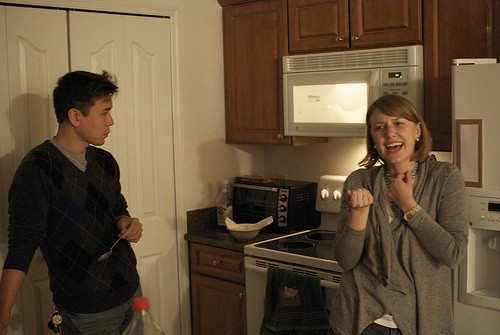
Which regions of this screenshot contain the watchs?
[404,205,422,221]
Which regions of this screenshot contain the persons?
[328,96,469,335]
[1,70,144,335]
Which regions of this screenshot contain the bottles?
[215,180,232,234]
[123,296,165,335]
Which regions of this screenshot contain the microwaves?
[281,45,423,138]
[231,178,321,235]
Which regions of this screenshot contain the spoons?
[97,236,121,261]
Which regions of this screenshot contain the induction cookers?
[244,175,349,272]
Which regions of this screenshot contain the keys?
[52,312,63,335]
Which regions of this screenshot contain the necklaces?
[385,164,419,215]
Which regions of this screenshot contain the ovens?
[244,256,341,335]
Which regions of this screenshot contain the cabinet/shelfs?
[188,243,246,335]
[218,0,500,152]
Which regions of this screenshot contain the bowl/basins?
[227,224,262,241]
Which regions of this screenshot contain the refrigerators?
[451,57,500,335]
